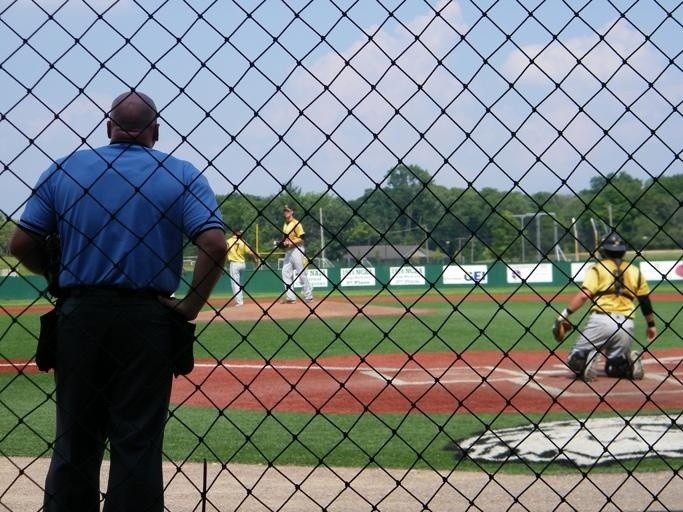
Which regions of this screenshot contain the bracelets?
[645,319,656,328]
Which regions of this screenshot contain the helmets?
[600,232,627,257]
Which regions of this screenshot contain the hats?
[232,229,244,234]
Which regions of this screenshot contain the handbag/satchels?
[173,318,196,377]
[36,308,54,372]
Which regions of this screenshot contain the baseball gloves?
[552,319,571,342]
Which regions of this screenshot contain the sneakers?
[626,349,645,380]
[280,299,314,304]
[232,301,245,306]
[582,348,603,383]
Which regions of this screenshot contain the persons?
[224,228,258,307]
[270,201,315,307]
[3,89,230,512]
[550,231,658,382]
